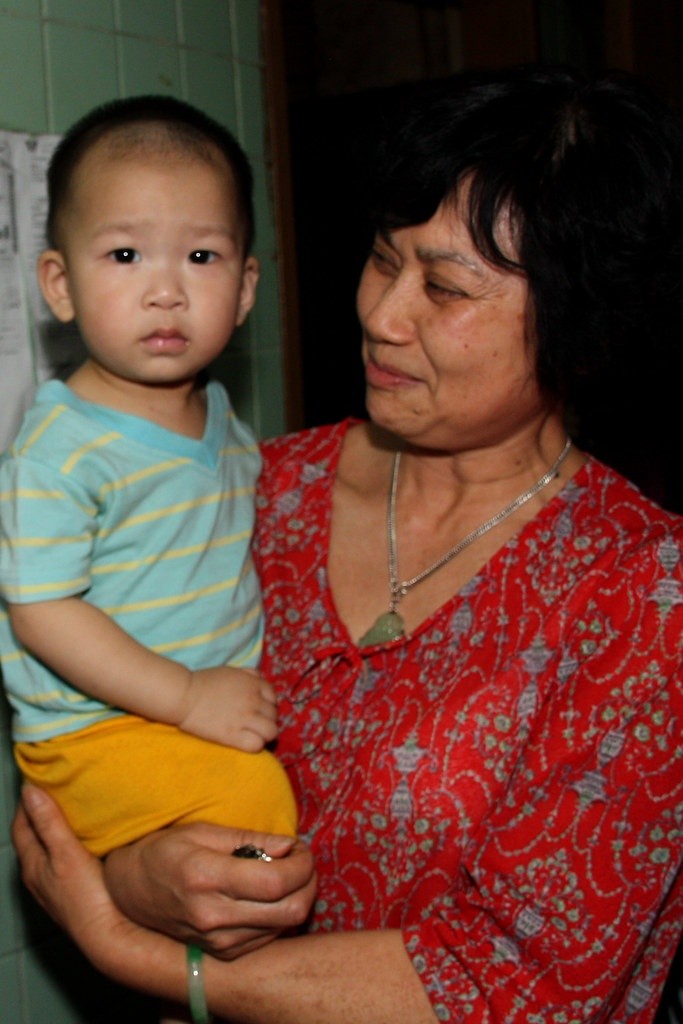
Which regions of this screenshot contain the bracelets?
[186,943,213,1024]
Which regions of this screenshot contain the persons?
[0,93,295,840]
[11,66,682,1024]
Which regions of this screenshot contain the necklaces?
[355,432,574,650]
